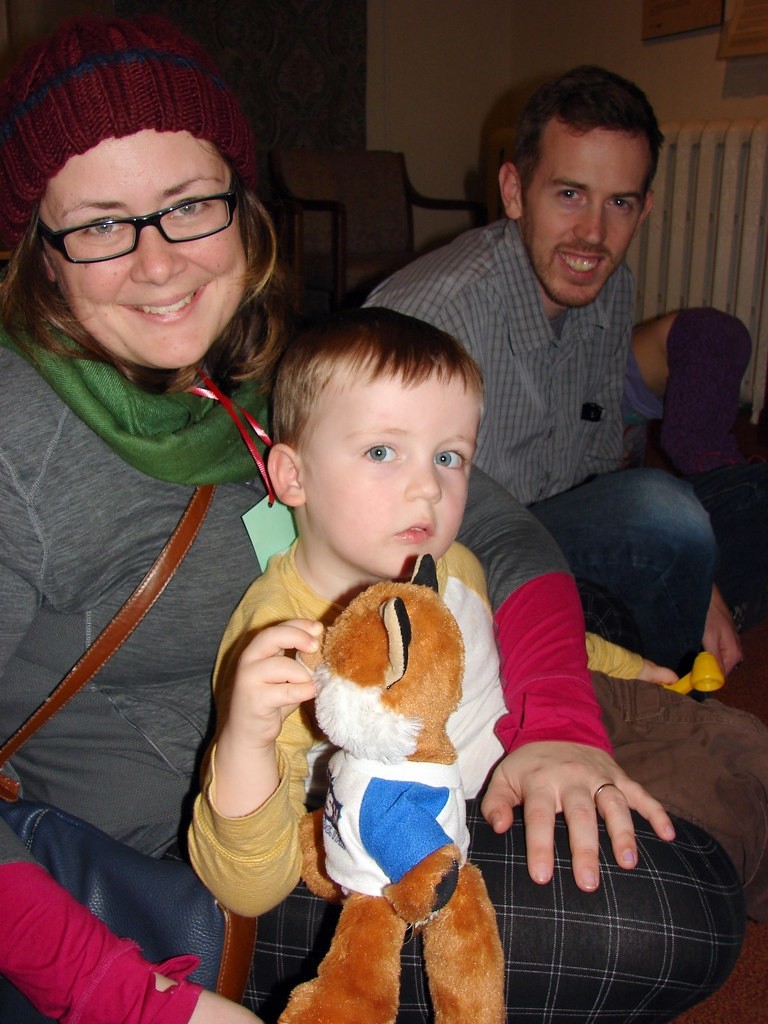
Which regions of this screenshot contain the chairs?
[268,145,488,325]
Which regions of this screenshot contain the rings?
[594,783,616,804]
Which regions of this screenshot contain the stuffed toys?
[276,553,506,1024]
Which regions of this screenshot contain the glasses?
[38,169,239,264]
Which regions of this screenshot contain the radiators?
[623,115,768,425]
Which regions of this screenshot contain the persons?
[362,64,753,703]
[188,307,679,917]
[0,4,745,1024]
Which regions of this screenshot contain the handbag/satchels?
[0,767,260,1002]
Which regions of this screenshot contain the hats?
[0,1,263,261]
